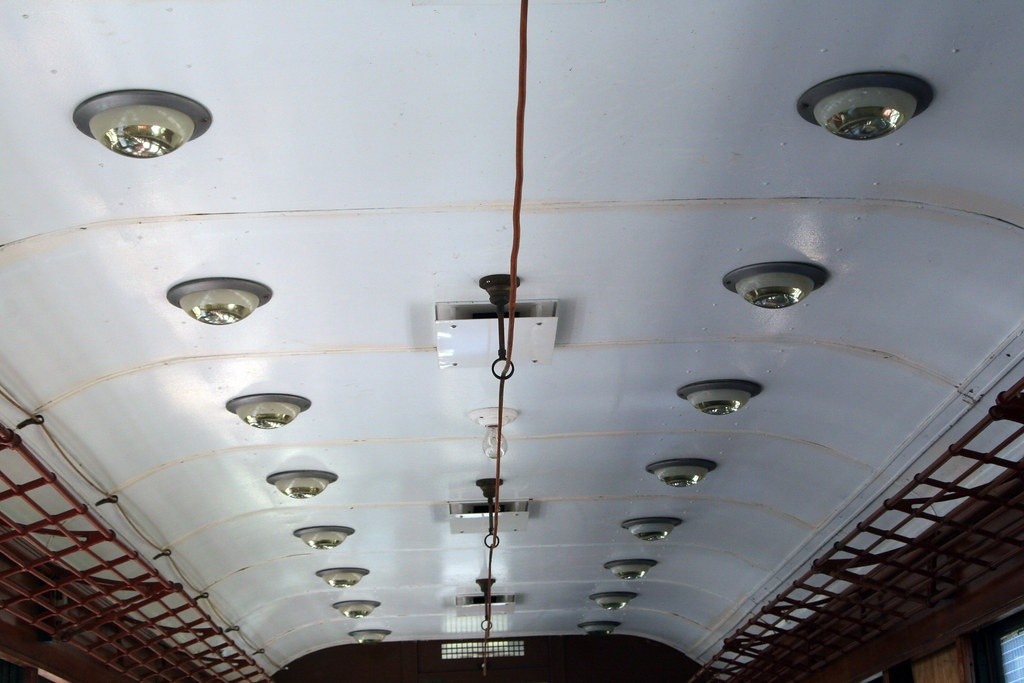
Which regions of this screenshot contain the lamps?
[620,517,684,541]
[453,594,516,616]
[432,299,560,370]
[796,71,934,141]
[348,629,392,644]
[677,378,764,416]
[166,276,273,326]
[722,262,831,310]
[315,567,370,589]
[645,458,718,488]
[266,470,339,499]
[226,393,312,430]
[448,500,532,533]
[589,591,639,611]
[293,525,356,551]
[604,558,659,580]
[578,621,622,637]
[332,599,381,619]
[72,88,212,159]
